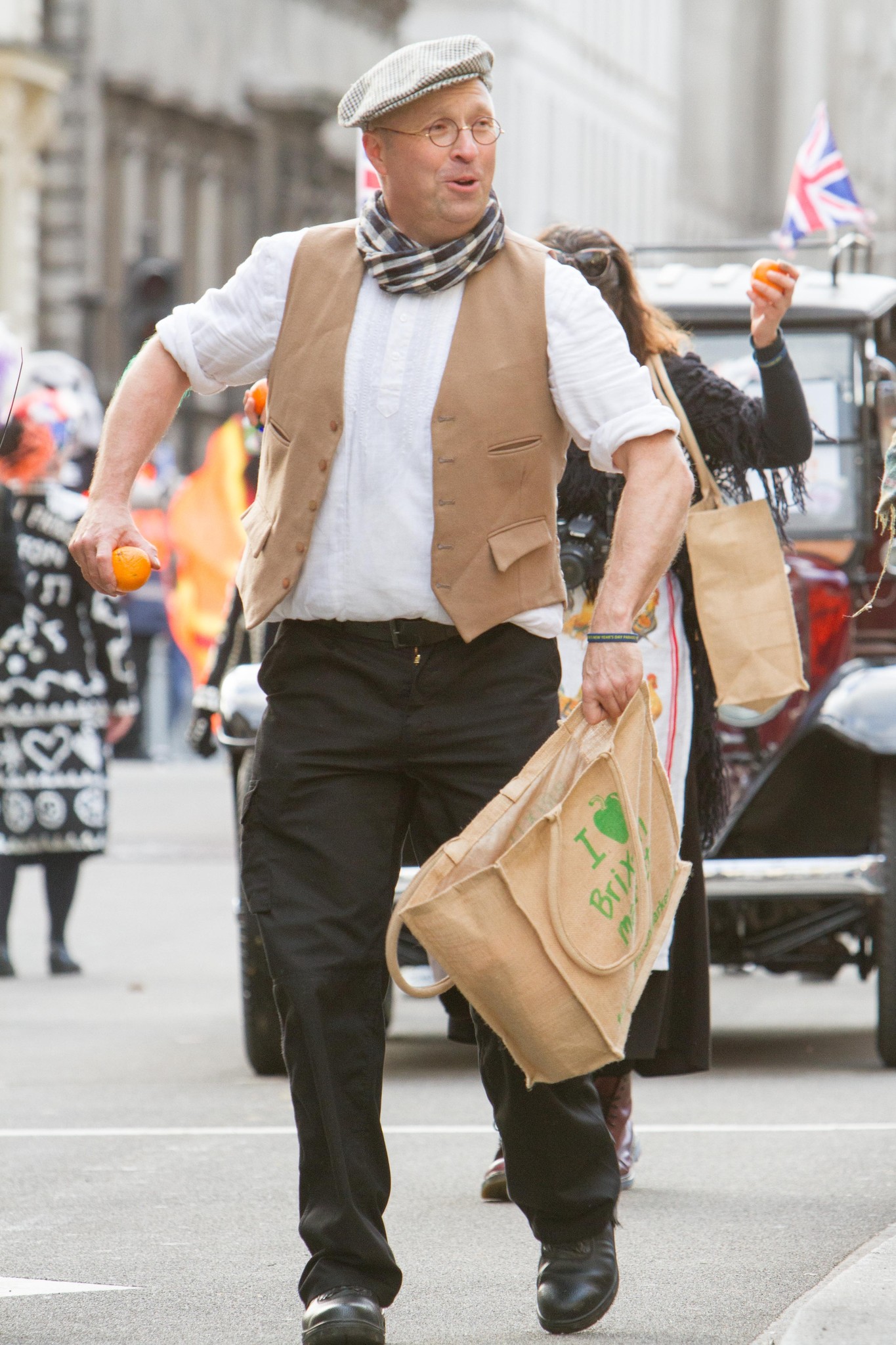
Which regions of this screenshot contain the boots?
[480,1145,512,1200]
[595,1066,641,1188]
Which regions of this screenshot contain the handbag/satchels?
[384,682,693,1089]
[646,354,810,715]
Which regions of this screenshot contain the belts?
[322,617,463,648]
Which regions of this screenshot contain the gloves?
[187,708,218,758]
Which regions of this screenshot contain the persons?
[481,228,813,1205]
[0,392,194,977]
[70,38,697,1345]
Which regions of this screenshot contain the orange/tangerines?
[751,258,787,298]
[112,546,151,592]
[249,377,269,414]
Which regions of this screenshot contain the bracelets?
[752,345,788,369]
[587,632,638,642]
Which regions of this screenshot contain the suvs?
[219,229,895,1081]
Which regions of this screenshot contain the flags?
[781,108,862,244]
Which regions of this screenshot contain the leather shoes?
[301,1285,386,1345]
[536,1207,620,1335]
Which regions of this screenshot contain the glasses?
[370,115,503,147]
[550,246,616,282]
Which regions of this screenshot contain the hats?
[0,395,67,486]
[339,34,496,130]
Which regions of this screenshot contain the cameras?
[555,513,609,591]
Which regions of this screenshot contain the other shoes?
[49,950,79,974]
[0,947,15,976]
